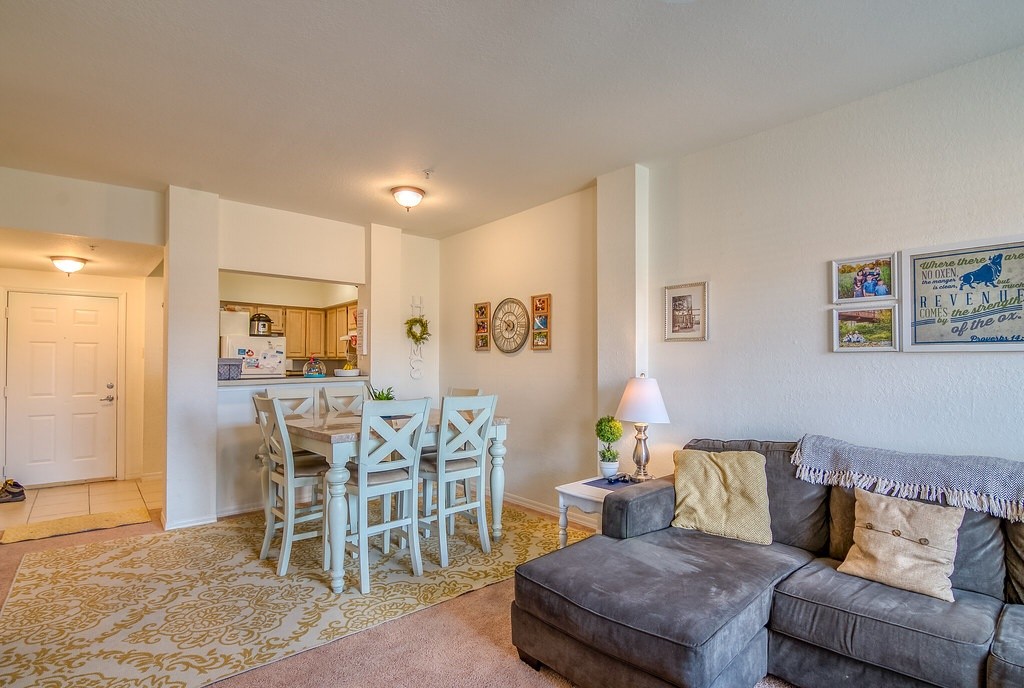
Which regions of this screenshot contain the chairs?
[423,386,483,538]
[346,397,431,594]
[322,385,367,417]
[418,394,498,567]
[252,395,330,576]
[266,387,322,524]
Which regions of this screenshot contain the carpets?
[0,507,152,544]
[0,485,596,688]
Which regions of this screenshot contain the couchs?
[511,434,828,688]
[767,434,1024,688]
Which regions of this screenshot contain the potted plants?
[371,386,397,419]
[595,416,623,477]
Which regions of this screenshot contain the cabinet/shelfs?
[285,310,325,360]
[326,302,357,359]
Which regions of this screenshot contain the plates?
[334,369,360,376]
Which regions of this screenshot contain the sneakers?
[0,480,26,502]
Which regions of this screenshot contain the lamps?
[391,186,425,211]
[49,257,87,277]
[614,372,671,482]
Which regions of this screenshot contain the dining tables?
[256,409,511,594]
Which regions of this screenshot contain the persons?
[843,330,864,343]
[853,266,888,297]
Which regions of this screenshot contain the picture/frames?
[832,304,899,351]
[664,281,707,342]
[531,294,551,350]
[902,234,1024,352]
[474,302,490,350]
[831,252,899,303]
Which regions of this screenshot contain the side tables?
[556,474,653,549]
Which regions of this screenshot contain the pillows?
[670,448,772,546]
[837,486,965,605]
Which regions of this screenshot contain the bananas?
[342,361,352,370]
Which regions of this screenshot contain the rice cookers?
[250,313,274,337]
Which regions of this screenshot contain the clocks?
[492,298,530,353]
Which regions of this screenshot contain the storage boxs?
[218,357,242,380]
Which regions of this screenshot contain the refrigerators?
[221,335,286,379]
